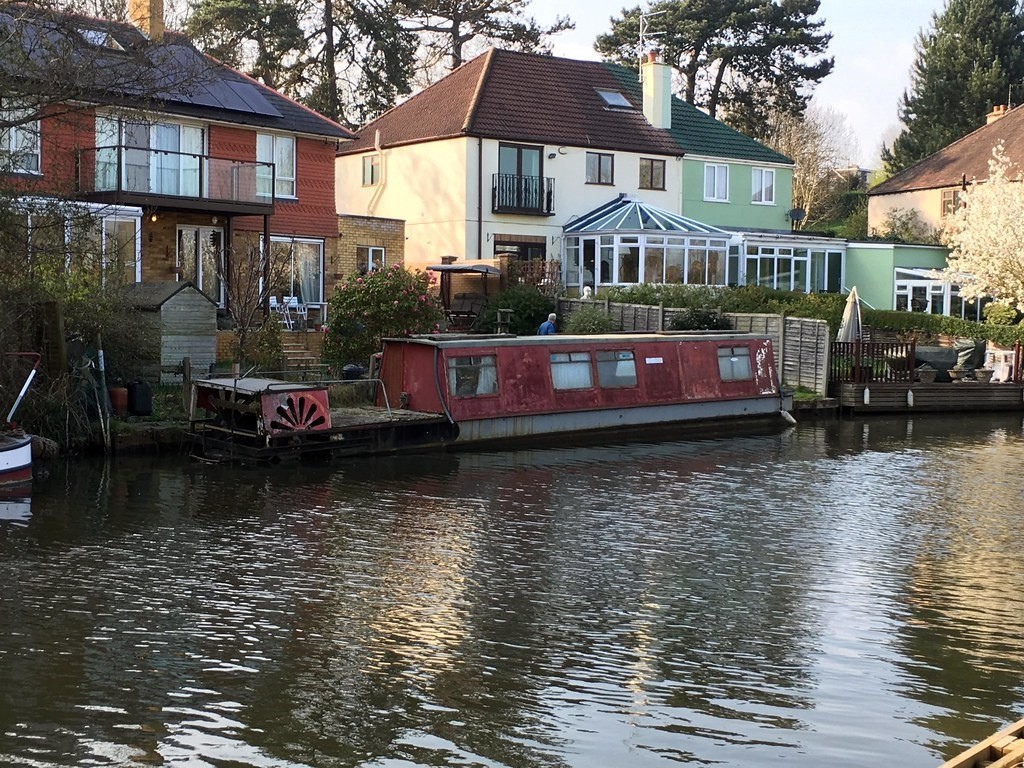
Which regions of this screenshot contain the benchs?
[443,293,485,328]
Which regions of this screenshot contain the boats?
[0,351,44,484]
[183,330,796,467]
[0,483,38,536]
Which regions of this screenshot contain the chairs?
[282,297,307,329]
[262,296,288,329]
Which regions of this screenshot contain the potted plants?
[974,366,995,383]
[947,364,969,383]
[915,365,938,383]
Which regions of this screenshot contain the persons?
[581,286,591,300]
[537,313,556,335]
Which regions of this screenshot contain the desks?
[301,302,331,324]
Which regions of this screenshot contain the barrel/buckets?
[852,364,873,382]
[314,324,321,331]
[109,377,152,421]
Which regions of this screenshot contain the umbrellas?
[833,285,858,365]
